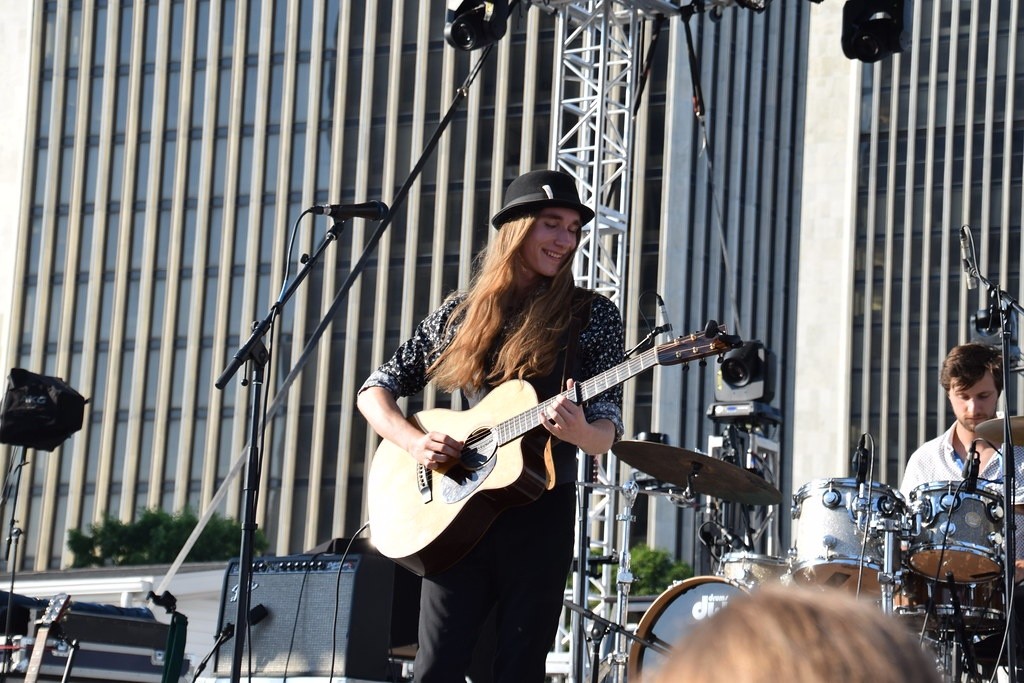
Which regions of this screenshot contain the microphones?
[959,229,977,290]
[308,200,389,221]
[222,604,267,642]
[658,295,674,344]
[852,435,866,473]
[713,521,743,550]
[962,441,978,478]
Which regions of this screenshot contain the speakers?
[213,553,395,682]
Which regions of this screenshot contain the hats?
[491,169,595,231]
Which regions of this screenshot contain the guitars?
[365,320,744,576]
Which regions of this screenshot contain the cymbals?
[1011,503,1024,515]
[969,415,1024,447]
[613,440,782,507]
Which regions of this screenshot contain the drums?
[791,479,907,593]
[903,479,1010,585]
[624,580,750,682]
[720,550,789,592]
[893,571,1006,634]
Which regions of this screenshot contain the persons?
[356,170,625,683]
[897,342,1024,683]
